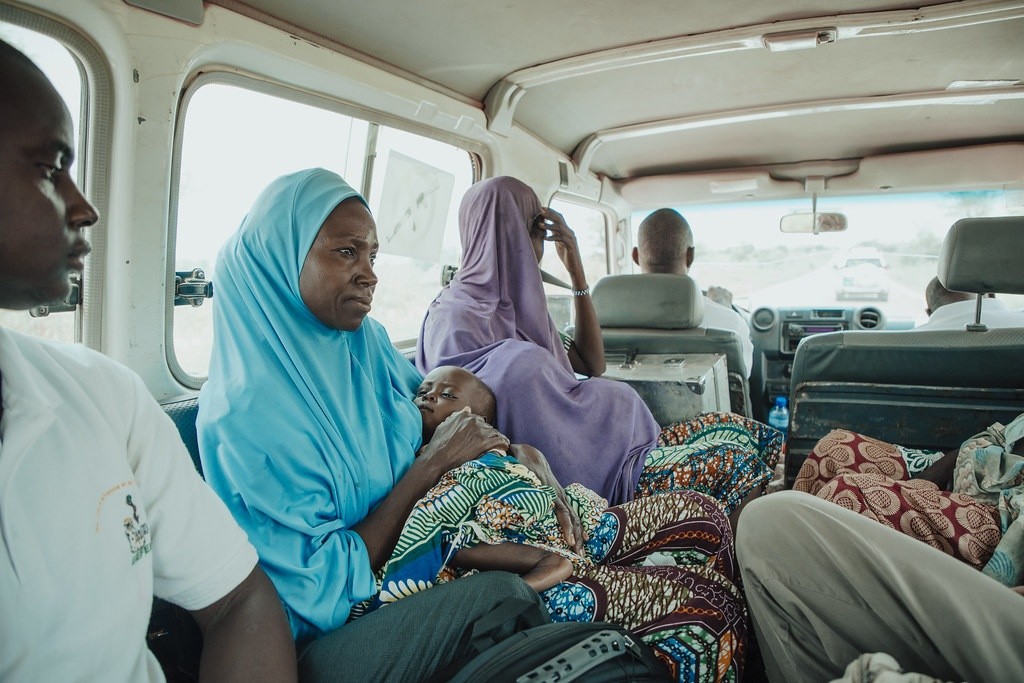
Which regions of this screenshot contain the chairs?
[562,272,756,416]
[786,215,1024,407]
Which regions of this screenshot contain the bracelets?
[571,284,589,296]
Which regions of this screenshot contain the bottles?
[767,397,789,442]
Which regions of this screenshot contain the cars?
[834,254,893,301]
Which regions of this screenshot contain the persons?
[0,39,547,683]
[734,272,1024,683]
[194,166,780,683]
